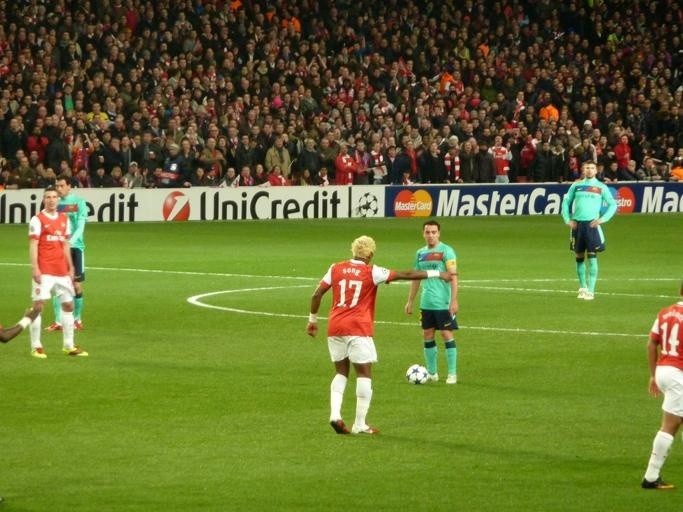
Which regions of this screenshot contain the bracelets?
[308,311,317,323]
[15,316,32,330]
[426,269,440,279]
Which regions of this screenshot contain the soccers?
[405,365,429,385]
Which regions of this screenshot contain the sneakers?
[577,288,594,300]
[427,373,439,382]
[642,476,675,489]
[63,346,87,357]
[32,348,47,359]
[330,418,349,433]
[445,374,457,385]
[351,423,378,435]
[47,320,82,331]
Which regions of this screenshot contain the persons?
[1,1,683,190]
[303,233,461,435]
[559,159,618,302]
[403,221,461,388]
[641,286,683,489]
[43,173,88,332]
[26,187,88,360]
[0,304,42,343]
